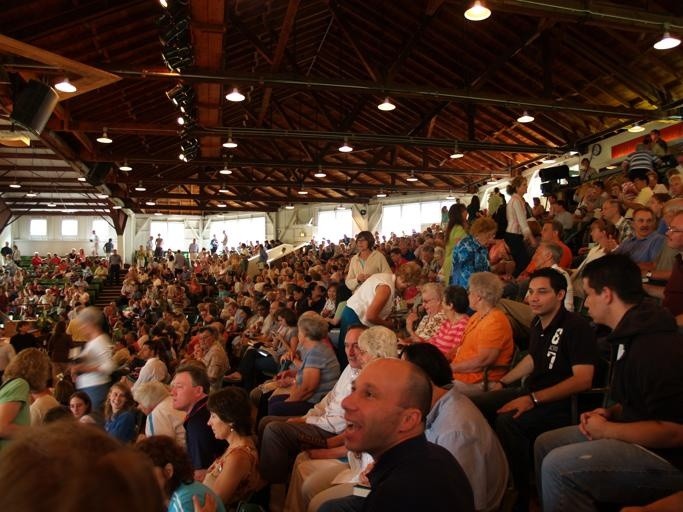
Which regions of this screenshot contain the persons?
[2,129,683,512]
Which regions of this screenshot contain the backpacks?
[493,202,509,240]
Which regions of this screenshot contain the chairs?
[186,314,199,327]
[569,341,612,424]
[240,349,276,385]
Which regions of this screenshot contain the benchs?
[19,256,106,305]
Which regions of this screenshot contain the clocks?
[592,144,601,156]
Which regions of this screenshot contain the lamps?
[10,181,21,189]
[653,31,680,51]
[155,0,199,164]
[517,110,535,124]
[406,174,419,181]
[54,74,156,207]
